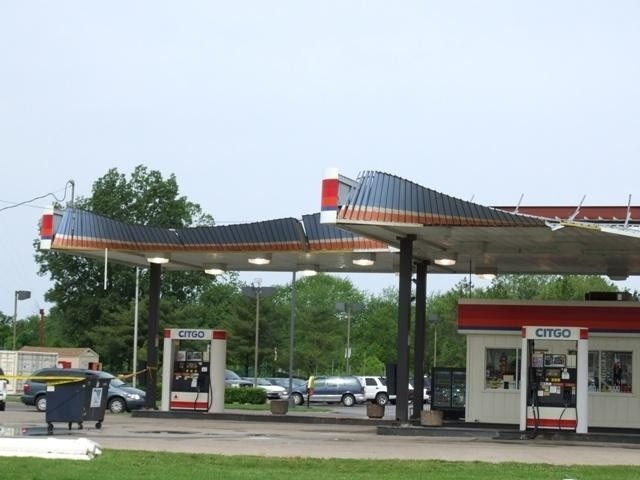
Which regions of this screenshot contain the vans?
[21,368,146,414]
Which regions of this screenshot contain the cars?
[485,368,519,389]
[588,370,632,391]
[433,384,466,407]
[224,369,431,406]
[0,368,9,411]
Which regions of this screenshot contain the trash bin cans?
[45,377,112,431]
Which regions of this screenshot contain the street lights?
[241,282,276,386]
[335,291,362,378]
[12,290,31,351]
[428,310,444,368]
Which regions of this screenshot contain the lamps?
[147,253,170,264]
[247,254,271,265]
[433,250,456,265]
[605,267,631,281]
[351,252,377,267]
[203,262,227,276]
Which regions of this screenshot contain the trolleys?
[46,370,116,431]
